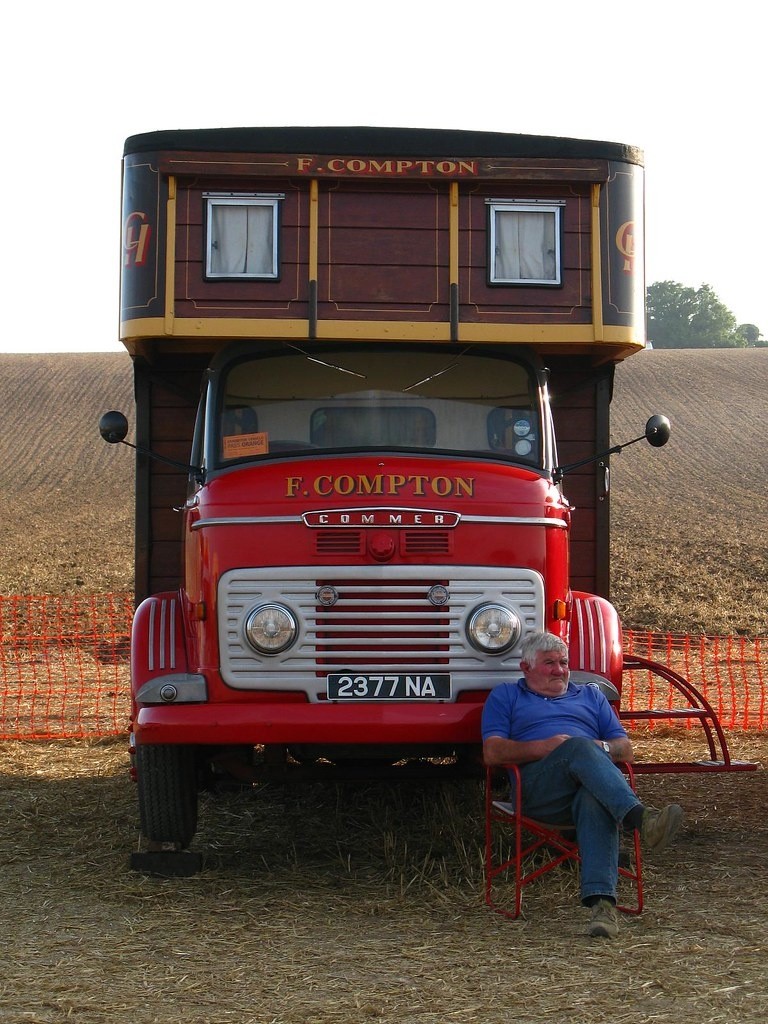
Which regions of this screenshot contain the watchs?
[600,740,610,753]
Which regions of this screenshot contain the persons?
[481,633,684,939]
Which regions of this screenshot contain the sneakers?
[589,899,619,939]
[641,804,685,855]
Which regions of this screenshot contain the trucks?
[100,125,672,851]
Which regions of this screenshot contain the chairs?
[484,760,644,920]
